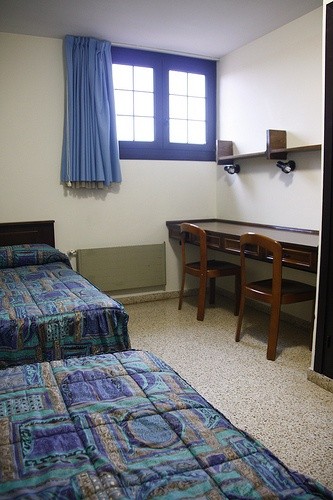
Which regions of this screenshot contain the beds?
[1,351,333,500]
[0,221,130,367]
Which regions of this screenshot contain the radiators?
[70,242,167,290]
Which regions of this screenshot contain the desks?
[166,218,319,272]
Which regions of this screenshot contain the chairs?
[178,223,241,321]
[236,233,316,361]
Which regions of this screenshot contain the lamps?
[277,160,296,174]
[224,164,240,174]
